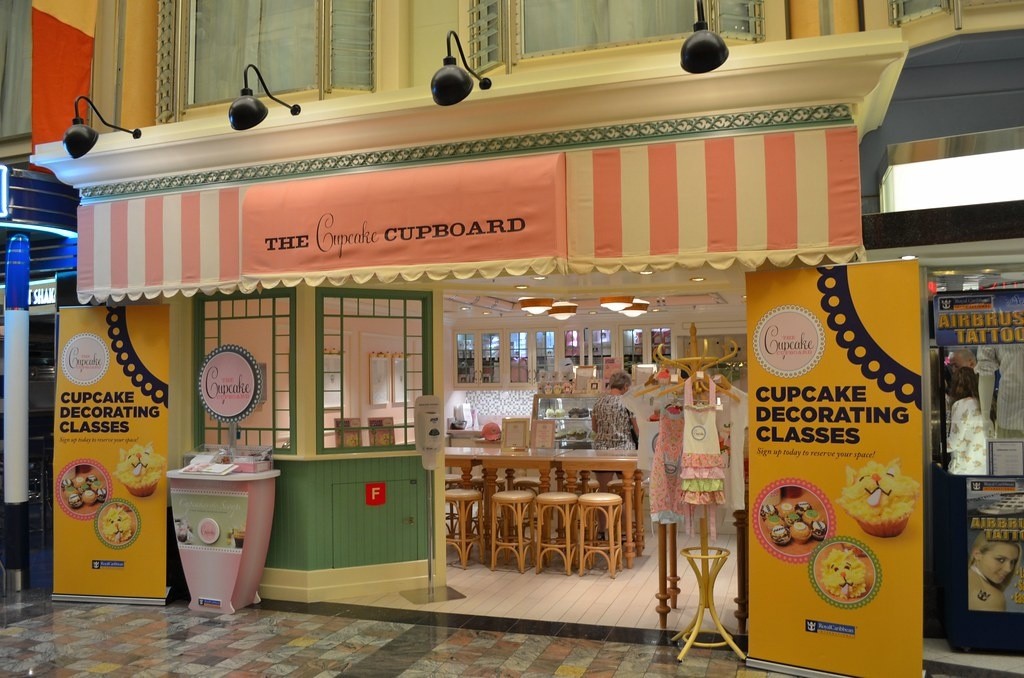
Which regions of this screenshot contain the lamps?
[517,295,553,315]
[680,0,728,73]
[598,294,633,311]
[618,297,651,317]
[63,95,142,158]
[228,64,301,130]
[547,301,578,320]
[429,29,492,106]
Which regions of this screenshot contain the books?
[183,462,235,473]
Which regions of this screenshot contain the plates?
[197,518,219,545]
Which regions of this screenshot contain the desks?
[557,447,636,568]
[442,444,571,568]
[165,469,281,612]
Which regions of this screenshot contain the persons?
[591,369,640,541]
[942,344,1024,475]
[968,530,1022,611]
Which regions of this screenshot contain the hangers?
[631,359,742,404]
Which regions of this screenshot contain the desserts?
[546,407,596,441]
[544,382,572,395]
[821,546,866,599]
[102,507,133,543]
[113,441,167,496]
[61,475,107,508]
[760,502,827,545]
[836,459,922,538]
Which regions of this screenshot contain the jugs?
[451,421,467,429]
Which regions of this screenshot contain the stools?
[443,475,645,579]
